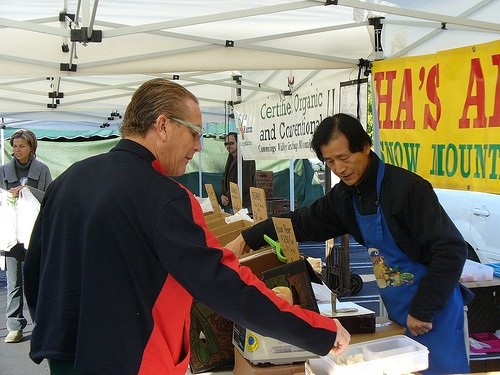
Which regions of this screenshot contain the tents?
[0,119,325,213]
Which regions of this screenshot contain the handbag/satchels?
[17,188,41,250]
[0,188,18,252]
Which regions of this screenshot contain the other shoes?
[4,330,23,343]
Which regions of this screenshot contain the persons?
[0,129,53,342]
[219,132,256,215]
[224,112,475,375]
[22,79,350,375]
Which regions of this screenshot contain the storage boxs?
[204,212,272,259]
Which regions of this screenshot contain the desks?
[233,316,424,375]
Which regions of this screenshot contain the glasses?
[224,142,236,146]
[169,116,204,139]
